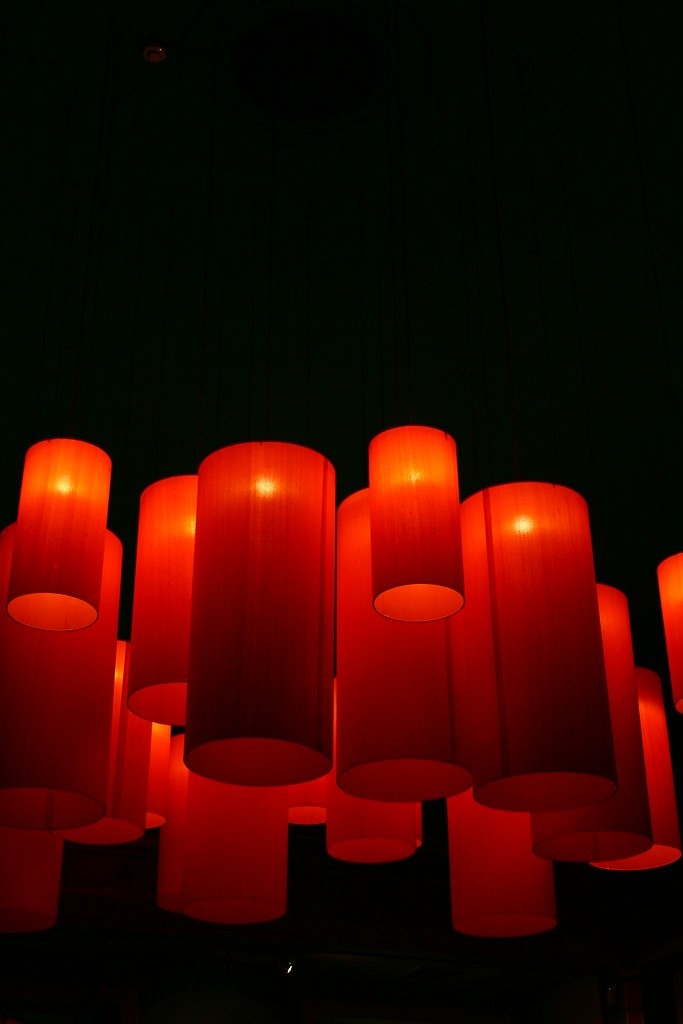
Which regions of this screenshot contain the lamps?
[1,428,682,941]
